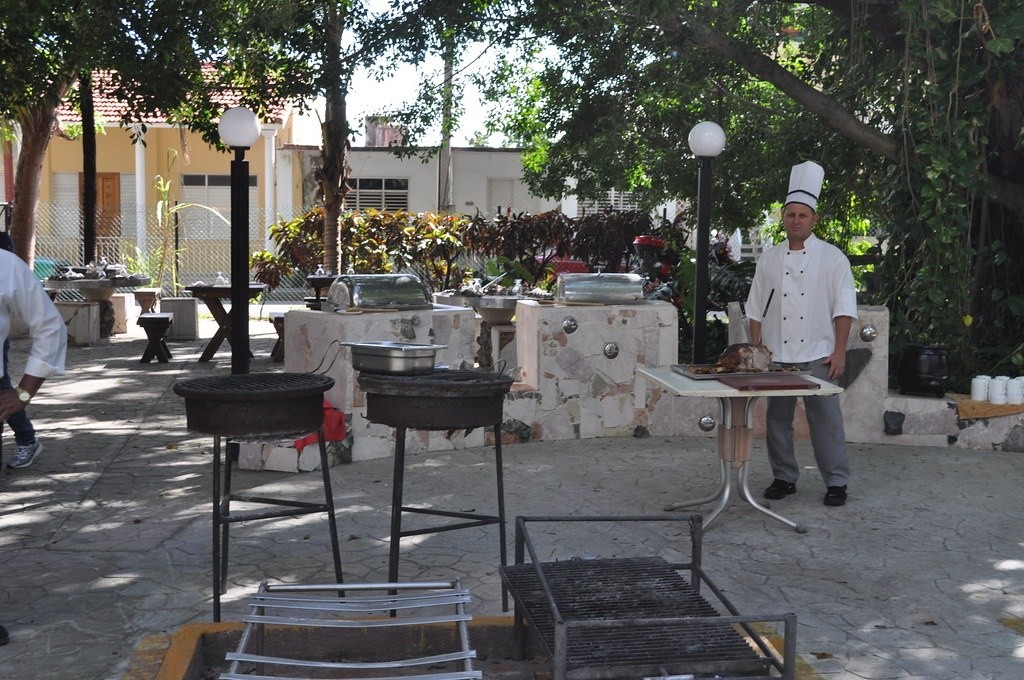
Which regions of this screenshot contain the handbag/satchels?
[295,400,345,452]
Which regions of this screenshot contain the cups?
[971,375,1024,404]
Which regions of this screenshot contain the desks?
[45,281,152,339]
[636,367,844,534]
[307,274,340,307]
[436,294,555,367]
[184,283,267,362]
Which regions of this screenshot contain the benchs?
[44,287,59,301]
[136,312,174,362]
[302,297,327,310]
[134,287,163,324]
[269,312,284,364]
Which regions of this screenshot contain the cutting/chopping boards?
[717,375,821,390]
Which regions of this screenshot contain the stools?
[55,300,102,345]
[111,293,135,333]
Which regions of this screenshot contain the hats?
[783,161,825,213]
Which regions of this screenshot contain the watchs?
[15,387,30,406]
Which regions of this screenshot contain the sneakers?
[764,479,796,499]
[7,439,43,469]
[824,484,847,506]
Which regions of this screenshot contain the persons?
[745,161,858,507]
[0,248,68,469]
